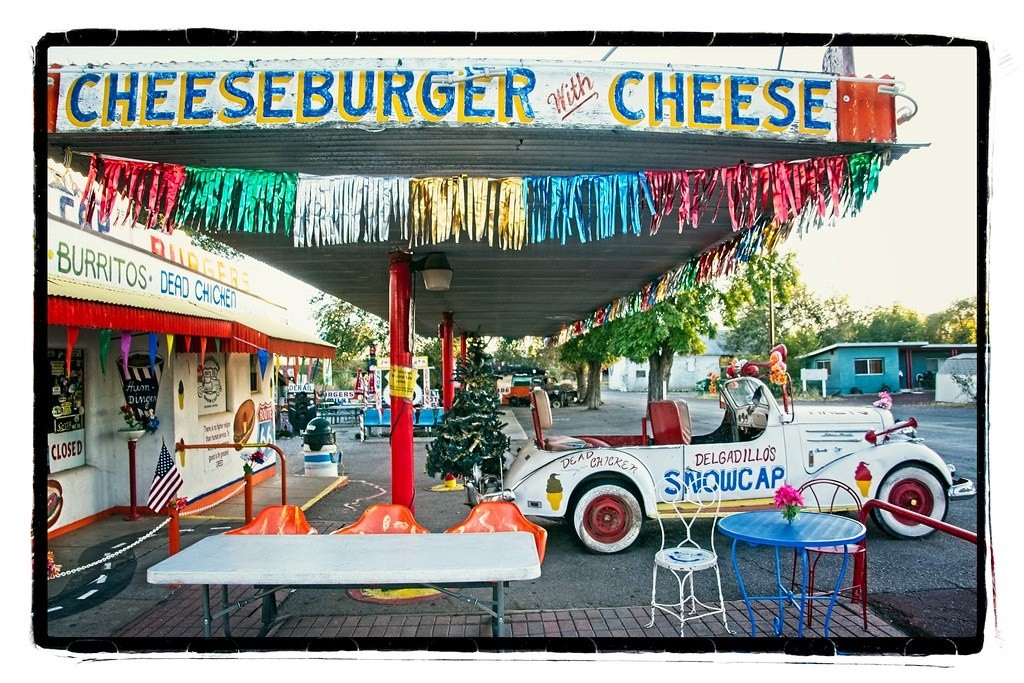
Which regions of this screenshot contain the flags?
[147,444,184,513]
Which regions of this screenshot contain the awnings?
[48,275,232,339]
[177,299,337,359]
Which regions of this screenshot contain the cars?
[466,344,977,556]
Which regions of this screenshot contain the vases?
[116,430,146,442]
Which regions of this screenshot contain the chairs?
[788,478,868,631]
[364,408,445,438]
[644,476,737,637]
[217,500,548,623]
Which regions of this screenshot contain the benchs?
[648,399,692,445]
[529,389,593,450]
[319,414,361,424]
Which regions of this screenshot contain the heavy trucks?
[505,376,580,409]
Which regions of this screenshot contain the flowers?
[241,450,265,474]
[769,362,788,386]
[774,485,805,524]
[119,404,155,431]
[166,496,188,511]
[706,357,738,394]
[873,390,892,410]
[47,551,62,579]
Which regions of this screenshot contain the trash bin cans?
[299,416,342,476]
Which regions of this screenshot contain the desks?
[147,533,541,637]
[717,510,863,637]
[317,402,365,427]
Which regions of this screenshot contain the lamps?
[412,253,454,291]
